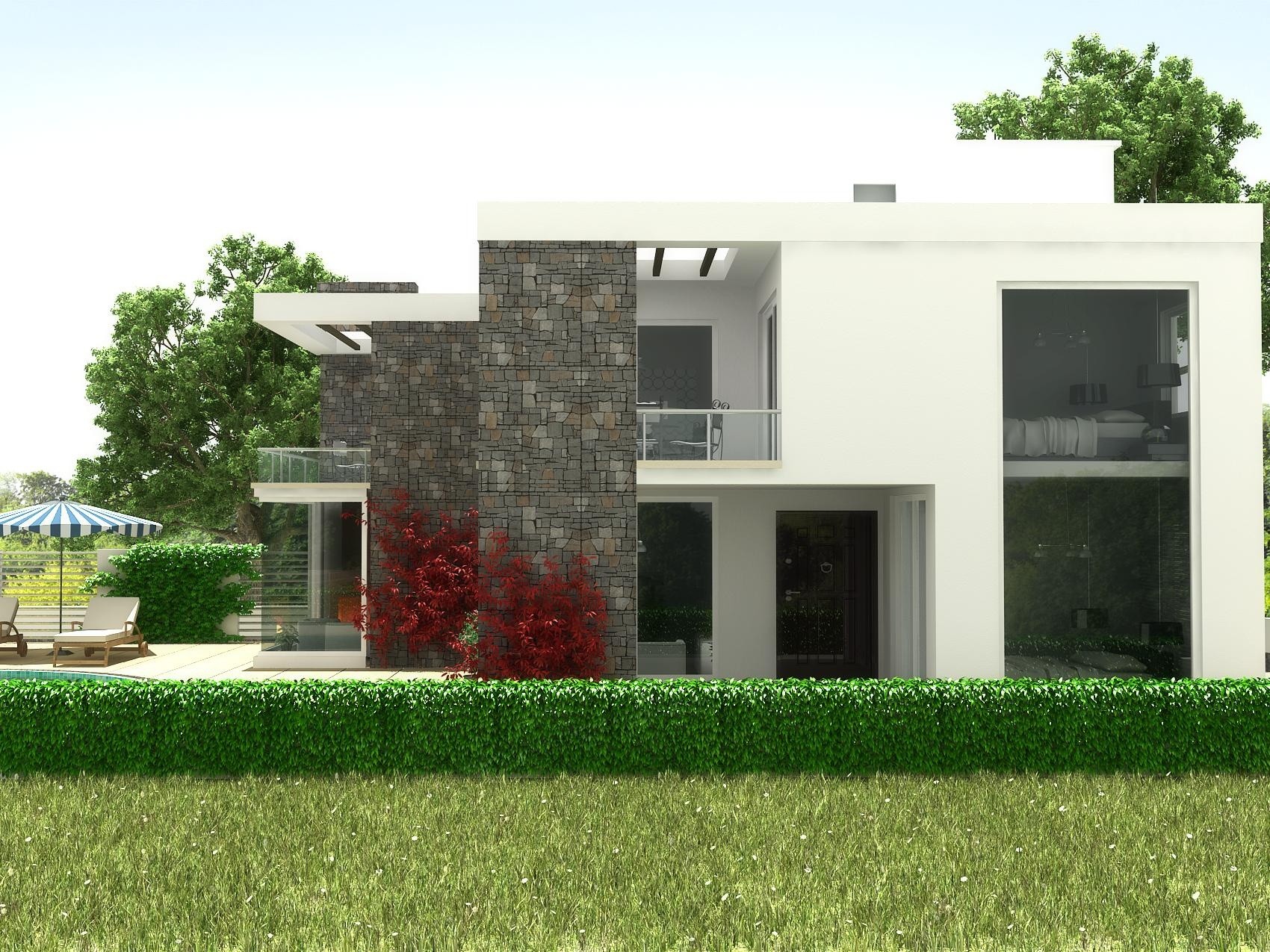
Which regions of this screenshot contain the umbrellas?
[0,501,164,649]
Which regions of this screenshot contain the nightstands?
[1147,442,1187,461]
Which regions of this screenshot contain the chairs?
[636,400,730,460]
[331,437,369,483]
[0,598,28,657]
[52,597,148,667]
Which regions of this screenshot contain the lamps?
[1137,291,1182,388]
[1034,291,1088,348]
[1035,484,1093,558]
[1069,290,1108,404]
[1142,478,1183,648]
[1072,480,1109,629]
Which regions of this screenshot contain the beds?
[1005,653,1152,679]
[1003,400,1170,459]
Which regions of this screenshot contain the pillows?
[1072,650,1147,672]
[1090,409,1144,422]
